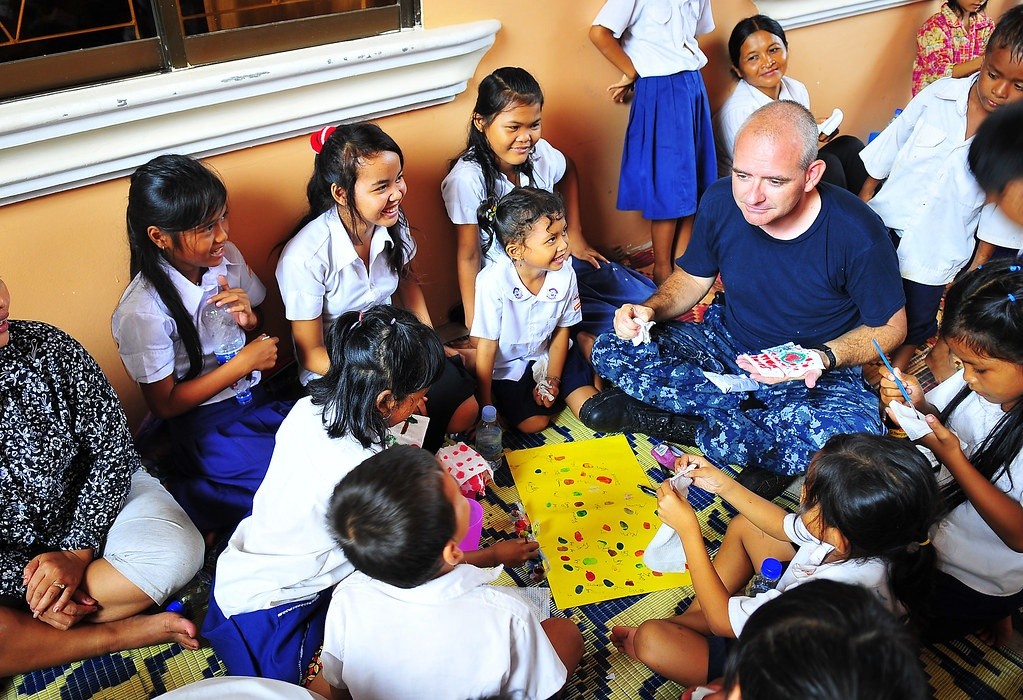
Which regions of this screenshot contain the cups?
[457,497,484,553]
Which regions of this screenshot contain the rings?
[54,583,65,589]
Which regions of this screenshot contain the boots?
[737,464,799,501]
[579,387,704,446]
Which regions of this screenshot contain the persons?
[711,15,882,198]
[610,433,939,694]
[469,185,599,434]
[110,153,291,514]
[0,277,206,677]
[275,123,479,435]
[912,0,996,99]
[318,445,584,700]
[725,579,924,700]
[879,257,1023,643]
[588,0,717,285]
[858,3,1023,373]
[440,66,657,339]
[579,99,908,477]
[201,303,539,695]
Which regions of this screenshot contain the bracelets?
[815,345,836,370]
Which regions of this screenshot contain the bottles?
[744,556,782,598]
[474,405,503,472]
[164,526,236,618]
[200,302,253,407]
[886,108,903,128]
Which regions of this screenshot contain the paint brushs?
[872,338,921,419]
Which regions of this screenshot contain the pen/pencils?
[637,484,656,497]
[523,536,530,545]
